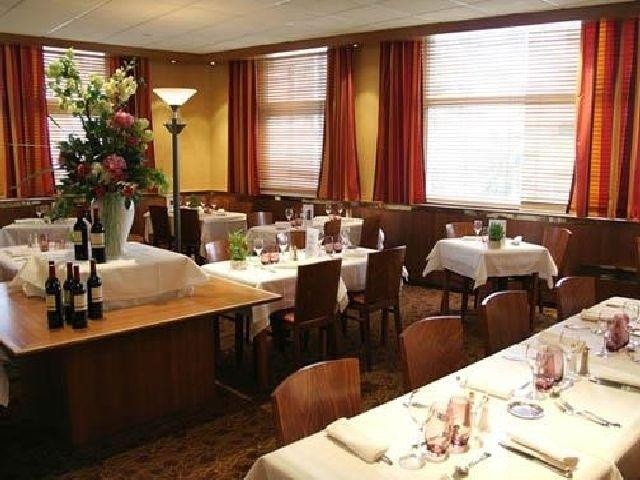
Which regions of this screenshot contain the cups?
[425,412,451,460]
[526,339,547,399]
[261,249,270,264]
[36,205,48,221]
[623,302,640,353]
[547,348,564,379]
[604,320,618,352]
[292,249,298,261]
[279,236,288,260]
[325,236,333,253]
[408,393,436,469]
[295,218,301,229]
[338,208,342,217]
[28,232,38,249]
[270,245,279,262]
[598,309,615,358]
[326,205,332,216]
[446,399,470,452]
[474,220,482,241]
[536,353,554,387]
[483,226,489,243]
[613,313,630,345]
[286,208,294,227]
[334,235,342,253]
[290,245,297,257]
[254,237,264,265]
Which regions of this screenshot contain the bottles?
[40,233,50,254]
[63,261,74,326]
[86,261,103,321]
[73,206,88,261]
[45,261,65,330]
[90,208,107,264]
[70,266,87,330]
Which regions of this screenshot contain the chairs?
[251,258,342,382]
[339,247,405,352]
[272,358,361,446]
[360,214,381,251]
[481,290,531,357]
[181,208,205,265]
[323,219,341,245]
[286,230,307,251]
[247,211,274,229]
[399,316,466,393]
[554,275,595,321]
[229,199,250,211]
[445,220,481,316]
[206,238,231,262]
[525,226,573,315]
[148,204,175,252]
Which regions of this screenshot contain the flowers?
[17,51,171,222]
[485,218,504,241]
[226,227,246,260]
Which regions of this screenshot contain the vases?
[488,236,501,249]
[100,192,134,264]
[231,257,245,269]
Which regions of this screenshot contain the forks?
[557,399,620,429]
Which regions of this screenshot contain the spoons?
[454,453,496,476]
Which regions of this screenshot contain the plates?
[506,400,545,420]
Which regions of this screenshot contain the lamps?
[153,87,197,106]
